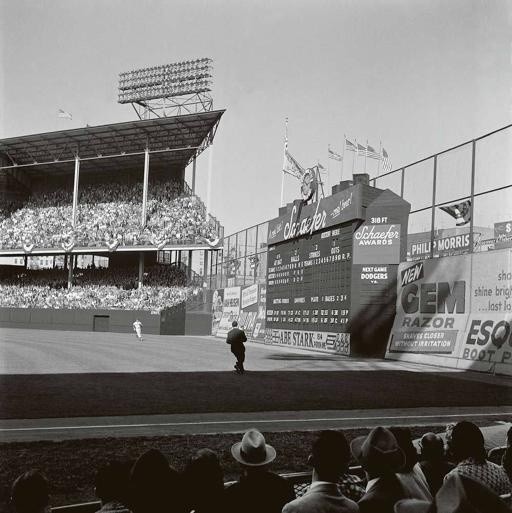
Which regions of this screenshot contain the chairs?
[487,445,508,465]
[495,493,512,513]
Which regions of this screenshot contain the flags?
[365,143,380,159]
[354,141,367,156]
[379,146,392,171]
[327,145,342,162]
[344,138,357,152]
[281,151,306,178]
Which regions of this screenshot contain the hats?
[350,428,407,474]
[232,431,276,467]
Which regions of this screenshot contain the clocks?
[298,168,315,201]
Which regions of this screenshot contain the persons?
[132,319,145,341]
[90,416,512,513]
[0,165,225,313]
[225,320,247,374]
[9,469,53,512]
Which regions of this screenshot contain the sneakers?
[234,365,244,374]
